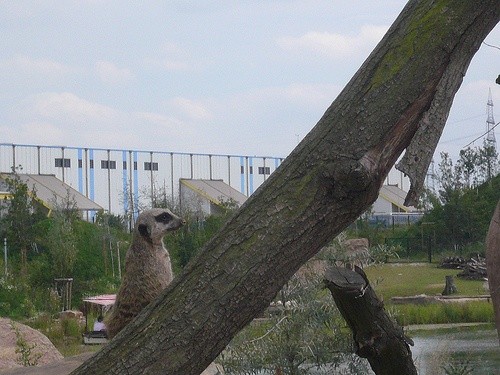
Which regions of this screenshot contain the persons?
[93,315,106,331]
